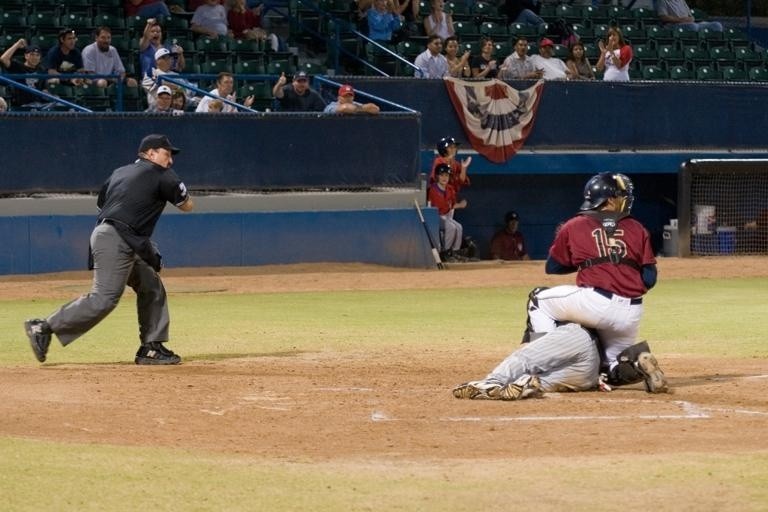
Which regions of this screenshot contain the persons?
[24,132,193,364]
[651,0,697,25]
[356,1,635,82]
[452,323,603,403]
[490,211,530,262]
[0,0,382,114]
[527,171,669,392]
[427,136,473,263]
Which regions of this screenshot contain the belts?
[594,286,641,305]
[98,218,114,224]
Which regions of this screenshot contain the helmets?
[437,135,461,156]
[435,163,452,175]
[580,172,635,215]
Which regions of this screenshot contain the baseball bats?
[414,198,444,270]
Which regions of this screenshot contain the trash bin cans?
[663,225,678,257]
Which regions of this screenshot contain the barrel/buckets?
[661,224,679,257]
[717,226,739,254]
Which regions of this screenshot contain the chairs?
[0,2,766,118]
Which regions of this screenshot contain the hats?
[338,85,355,96]
[156,85,171,96]
[539,38,554,48]
[506,212,519,222]
[294,71,308,83]
[155,48,178,61]
[26,45,42,55]
[139,135,181,155]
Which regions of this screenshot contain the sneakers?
[24,318,51,362]
[637,351,669,394]
[452,375,542,400]
[135,345,180,365]
